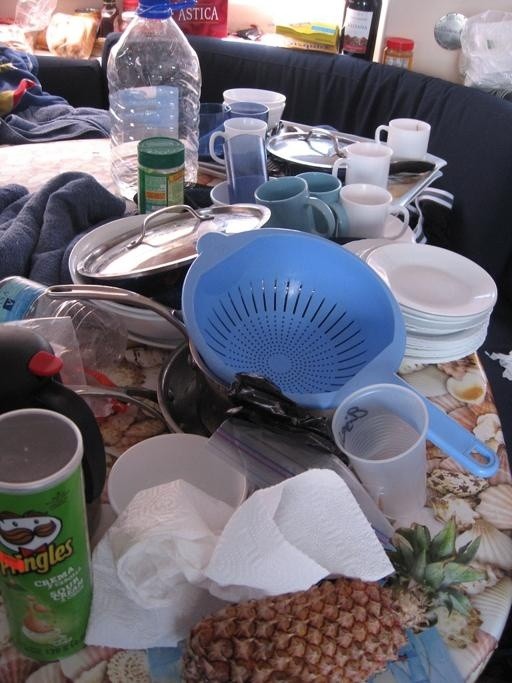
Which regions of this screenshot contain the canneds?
[119,11,137,32]
[381,38,413,72]
[74,8,100,38]
[137,137,186,214]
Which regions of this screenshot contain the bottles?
[105,0,203,202]
[101,0,121,37]
[1,273,129,372]
[338,0,382,61]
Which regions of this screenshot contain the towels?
[84,469,396,648]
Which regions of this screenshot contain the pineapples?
[181,518,486,683]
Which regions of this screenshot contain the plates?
[358,215,417,245]
[105,432,246,532]
[333,236,498,363]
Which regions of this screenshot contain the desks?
[1,139,511,682]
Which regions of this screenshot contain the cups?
[196,100,222,136]
[221,100,432,241]
[328,382,431,522]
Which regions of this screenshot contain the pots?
[68,203,272,349]
[46,282,389,483]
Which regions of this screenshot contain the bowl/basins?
[221,86,287,134]
[208,175,280,208]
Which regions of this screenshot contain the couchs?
[102,31,512,471]
[0,53,102,145]
